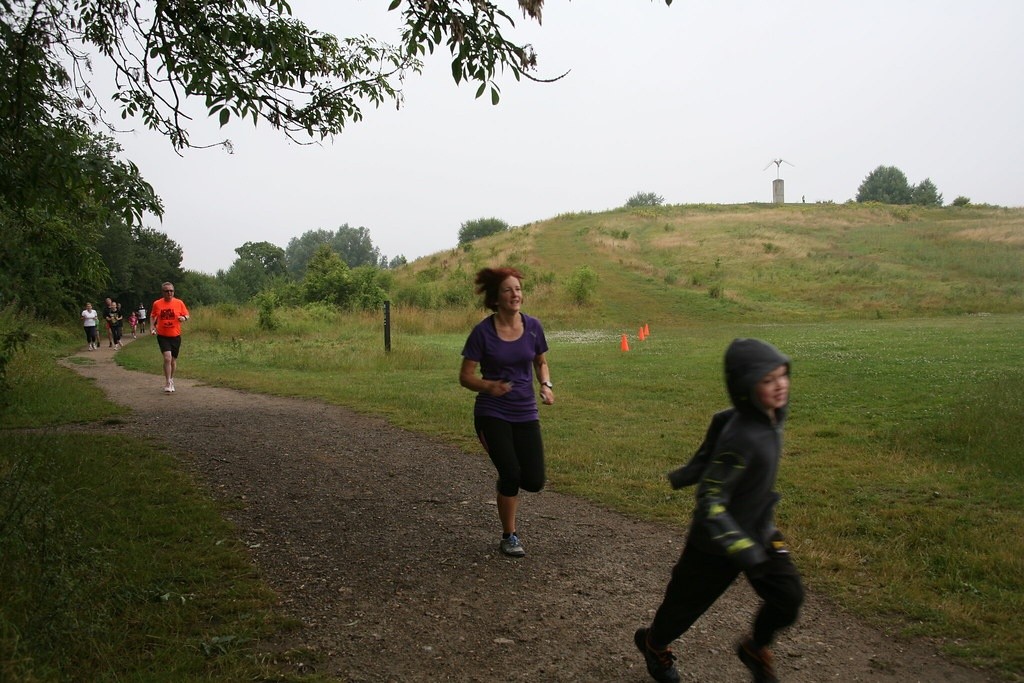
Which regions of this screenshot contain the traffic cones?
[639,326,645,341]
[644,322,650,337]
[620,333,629,352]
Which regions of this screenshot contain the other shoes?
[97,342,100,347]
[114,344,119,350]
[92,344,97,349]
[134,335,136,338]
[88,345,93,351]
[118,340,123,347]
[109,342,112,347]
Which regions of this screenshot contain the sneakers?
[738,636,780,683]
[501,532,525,557]
[634,627,679,683]
[164,379,175,392]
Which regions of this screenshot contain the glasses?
[163,289,175,292]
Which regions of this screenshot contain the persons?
[91,306,100,347]
[150,282,190,393]
[102,298,113,347]
[459,266,555,556]
[81,302,98,351]
[634,339,807,683]
[105,302,123,350]
[136,302,148,333]
[128,312,138,338]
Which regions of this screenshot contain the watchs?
[184,315,188,321]
[541,382,553,390]
